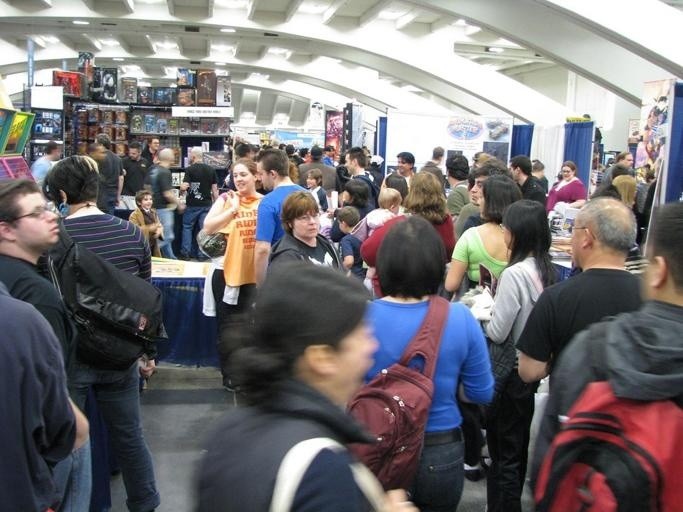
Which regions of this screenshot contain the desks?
[149,253,217,370]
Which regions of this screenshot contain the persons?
[29,135,655,478]
[0,282,92,512]
[527,200,682,512]
[192,261,419,512]
[344,217,494,512]
[39,154,160,512]
[476,200,562,512]
[0,177,92,512]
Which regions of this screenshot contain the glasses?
[293,213,319,221]
[567,225,597,241]
[7,201,57,224]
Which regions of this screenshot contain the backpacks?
[531,378,683,512]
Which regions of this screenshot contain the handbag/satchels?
[46,241,164,371]
[195,226,229,259]
[343,362,437,498]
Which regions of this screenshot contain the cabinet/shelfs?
[20,105,65,165]
[73,103,234,220]
